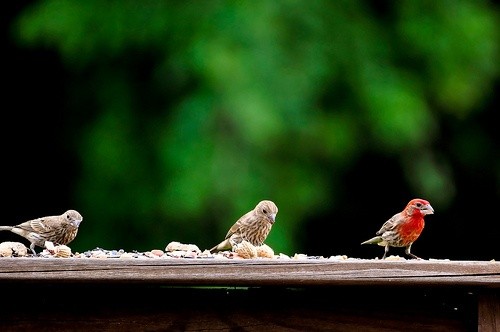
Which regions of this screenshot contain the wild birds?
[361,199,435,261]
[0,210,83,256]
[208,200,278,252]
